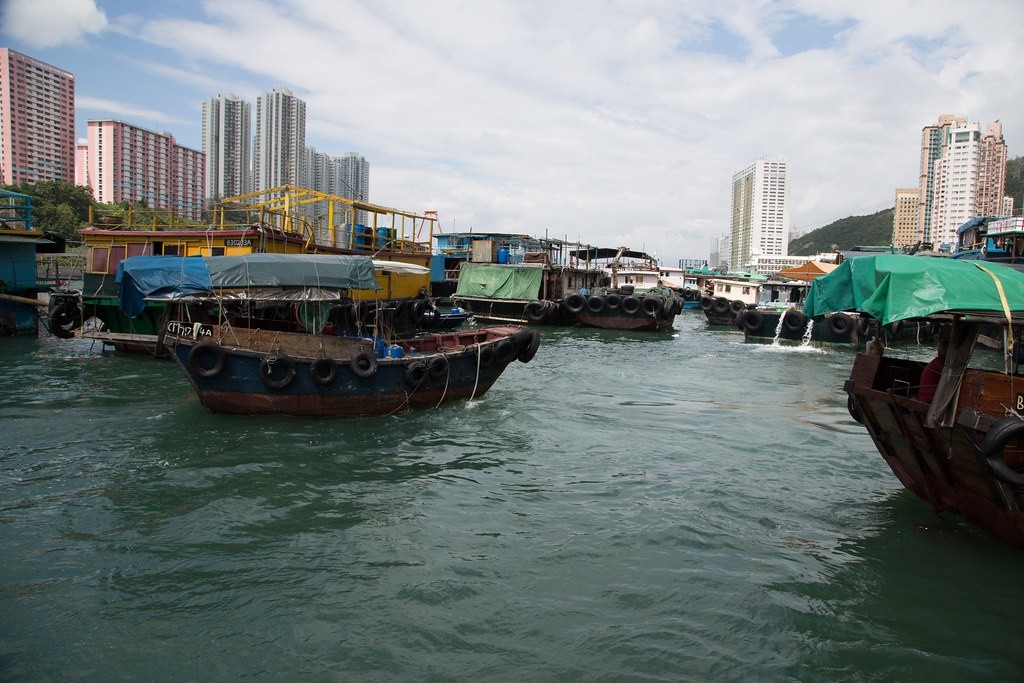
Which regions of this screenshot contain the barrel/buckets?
[373,338,386,360]
[497,248,507,264]
[452,307,460,314]
[381,342,405,357]
[334,223,389,250]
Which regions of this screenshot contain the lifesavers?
[519,328,541,365]
[428,356,450,380]
[259,352,296,387]
[527,300,546,321]
[493,340,514,367]
[187,342,227,378]
[310,355,338,385]
[783,310,805,332]
[668,286,701,300]
[699,298,744,314]
[741,310,764,331]
[982,418,1024,487]
[565,292,662,316]
[857,317,905,340]
[352,351,381,378]
[477,346,493,368]
[407,362,428,386]
[50,301,84,339]
[828,313,853,335]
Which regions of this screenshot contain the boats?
[0,184,540,417]
[805,255,1024,549]
[428,213,1024,353]
